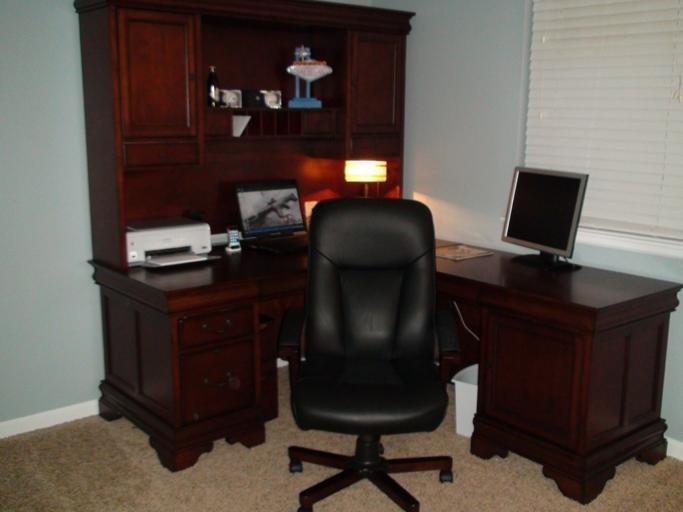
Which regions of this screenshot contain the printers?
[124,216,212,269]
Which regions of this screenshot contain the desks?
[98,229,340,476]
[450,241,670,504]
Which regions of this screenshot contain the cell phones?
[226,227,240,248]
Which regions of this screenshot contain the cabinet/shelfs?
[206,3,344,161]
[76,5,212,218]
[342,2,403,160]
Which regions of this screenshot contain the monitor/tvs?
[233,178,306,255]
[502,167,588,267]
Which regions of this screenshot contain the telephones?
[225,225,242,255]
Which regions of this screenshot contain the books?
[434,243,494,261]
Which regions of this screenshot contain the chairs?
[276,198,467,511]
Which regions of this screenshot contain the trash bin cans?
[451,364,479,438]
